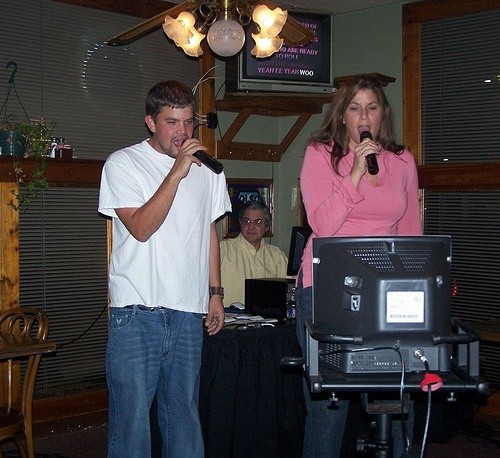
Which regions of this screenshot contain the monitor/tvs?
[310,235,453,374]
[236,10,335,94]
[287,226,313,276]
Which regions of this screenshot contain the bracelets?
[209,286,224,300]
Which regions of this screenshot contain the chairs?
[0,307,49,458]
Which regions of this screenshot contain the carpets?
[0,423,107,458]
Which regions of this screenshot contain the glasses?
[240,218,268,227]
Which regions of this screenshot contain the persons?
[296,75,424,458]
[98,81,234,458]
[220,200,288,308]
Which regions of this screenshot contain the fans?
[105,0,315,47]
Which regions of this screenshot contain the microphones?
[360,130,379,175]
[182,140,224,175]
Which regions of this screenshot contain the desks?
[150,310,490,458]
[0,332,56,360]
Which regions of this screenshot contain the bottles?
[50,136,71,157]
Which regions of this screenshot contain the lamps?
[163,0,288,58]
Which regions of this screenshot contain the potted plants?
[0,114,73,211]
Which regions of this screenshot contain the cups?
[55,146,73,158]
[1,130,23,156]
